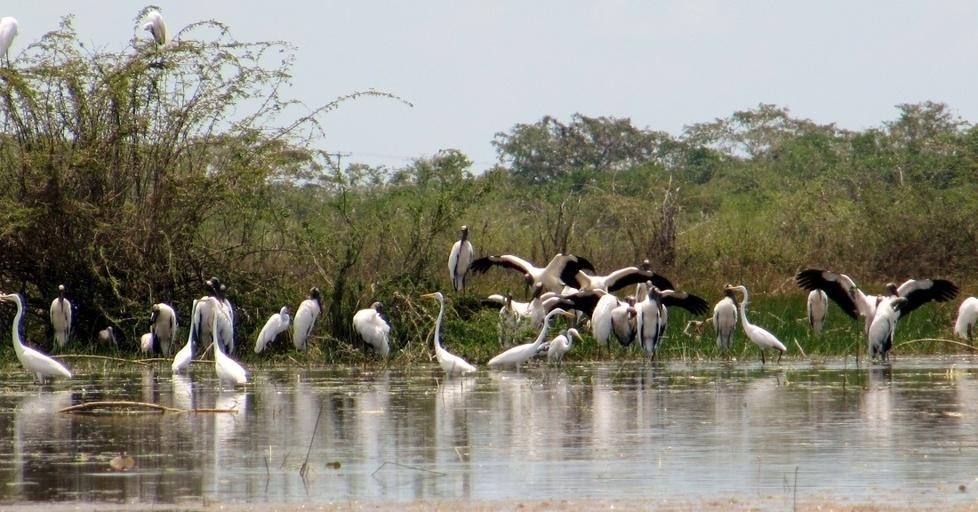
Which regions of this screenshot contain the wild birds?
[0,223,972,386]
